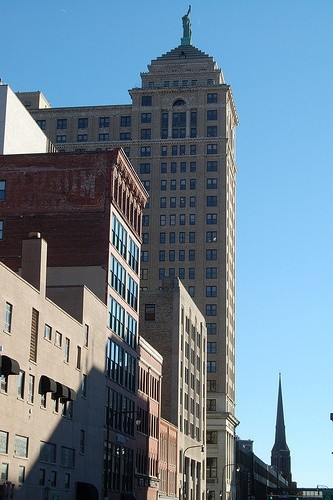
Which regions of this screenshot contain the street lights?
[182,444,205,499]
[220,463,241,500]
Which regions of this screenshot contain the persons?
[181,4,193,38]
